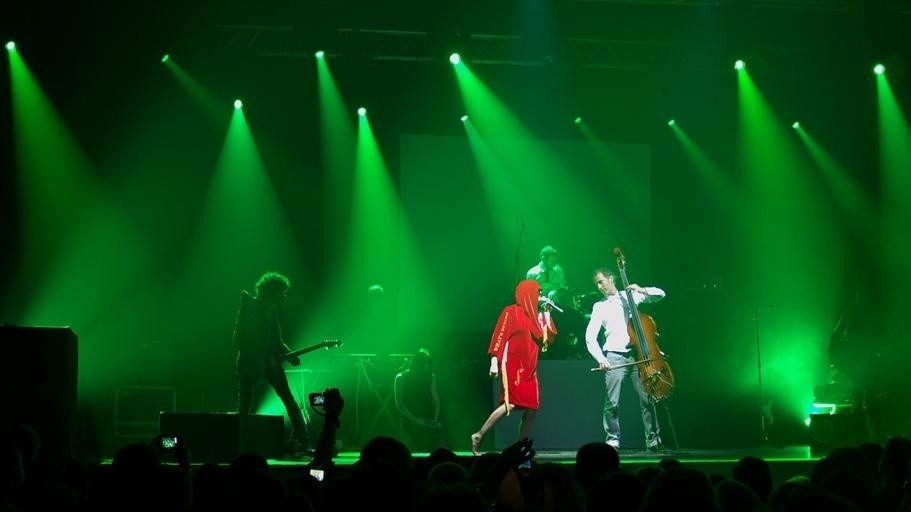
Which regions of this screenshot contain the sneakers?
[645,444,675,455]
[471,431,483,457]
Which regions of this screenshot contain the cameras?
[309,394,325,406]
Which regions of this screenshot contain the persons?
[471,279,558,456]
[233,272,307,443]
[394,348,446,451]
[526,245,565,297]
[585,267,672,454]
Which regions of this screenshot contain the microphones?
[541,296,564,313]
[520,215,527,236]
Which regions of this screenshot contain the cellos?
[613,248,676,403]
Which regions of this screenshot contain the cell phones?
[518,446,531,469]
[309,466,325,481]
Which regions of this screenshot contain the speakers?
[160,434,177,448]
[809,413,865,458]
[0,324,79,473]
[159,411,285,465]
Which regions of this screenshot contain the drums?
[547,288,583,320]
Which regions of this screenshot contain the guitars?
[238,339,343,389]
